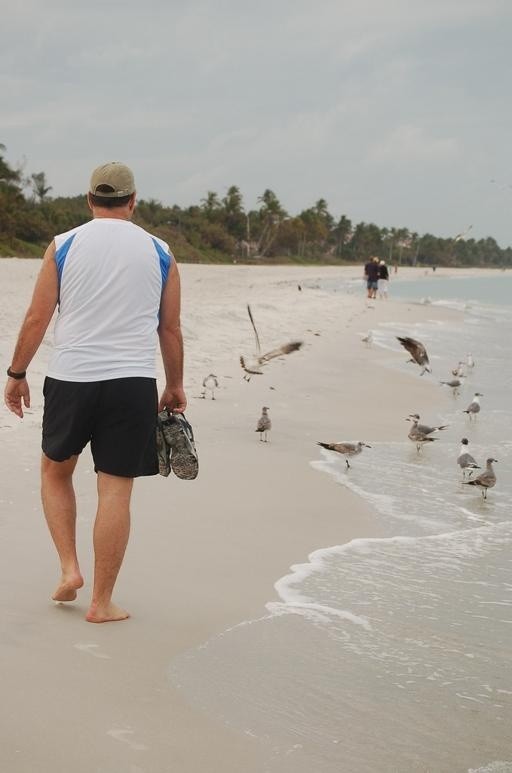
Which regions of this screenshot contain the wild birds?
[463,393,484,422]
[362,332,373,348]
[201,374,218,400]
[457,438,478,478]
[440,352,476,396]
[461,457,499,500]
[255,406,271,442]
[240,304,302,383]
[404,414,451,454]
[395,336,432,376]
[314,441,372,468]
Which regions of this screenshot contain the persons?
[364,255,390,301]
[3,160,188,623]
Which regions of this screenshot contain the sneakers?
[155,408,199,480]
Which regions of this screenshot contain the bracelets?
[6,366,26,379]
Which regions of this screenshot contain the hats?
[89,161,136,197]
